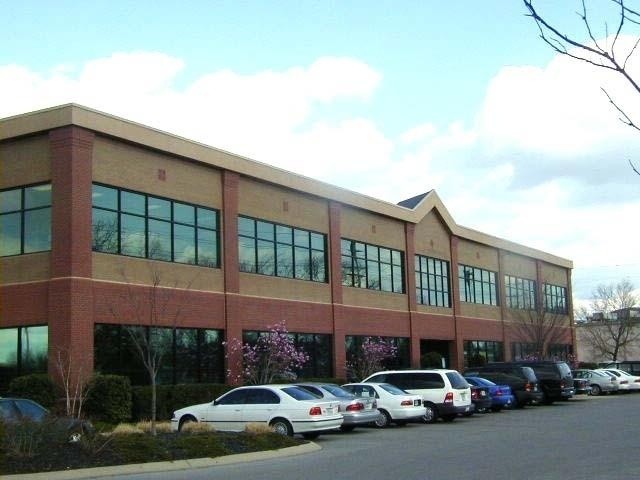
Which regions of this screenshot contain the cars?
[0,397,95,443]
